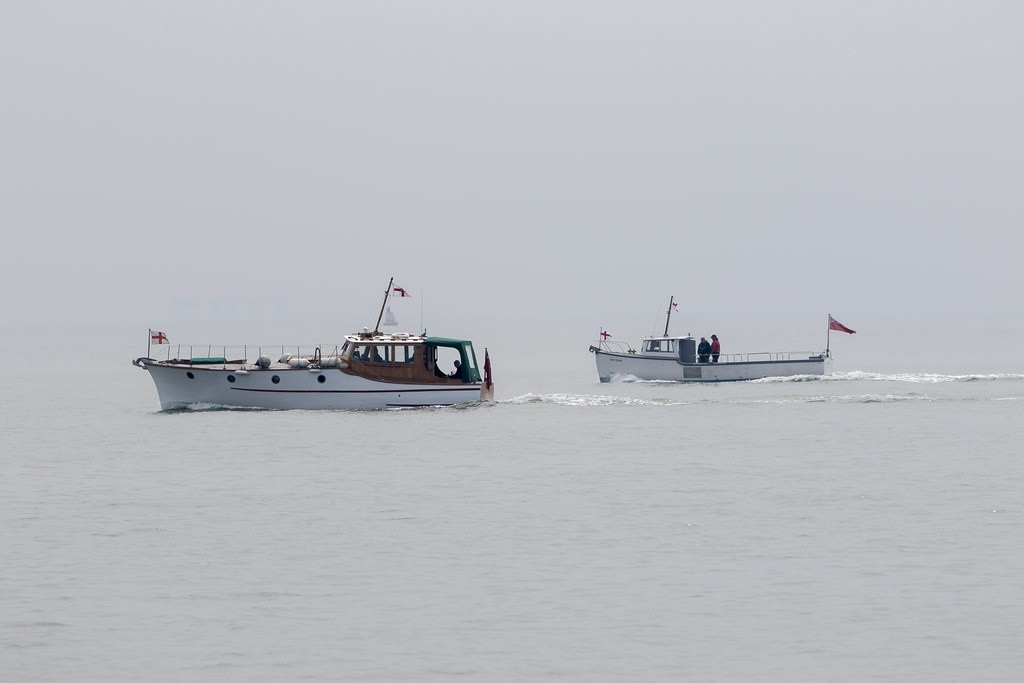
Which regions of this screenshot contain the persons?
[448,360,461,379]
[710,335,720,362]
[374,346,383,362]
[698,337,711,363]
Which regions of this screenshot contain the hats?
[710,335,716,338]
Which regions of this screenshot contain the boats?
[589,295,832,384]
[132,277,494,412]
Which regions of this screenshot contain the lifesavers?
[391,332,410,339]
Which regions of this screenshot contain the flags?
[484,350,491,390]
[151,331,170,344]
[601,330,612,341]
[673,302,679,311]
[393,283,411,297]
[830,317,856,334]
